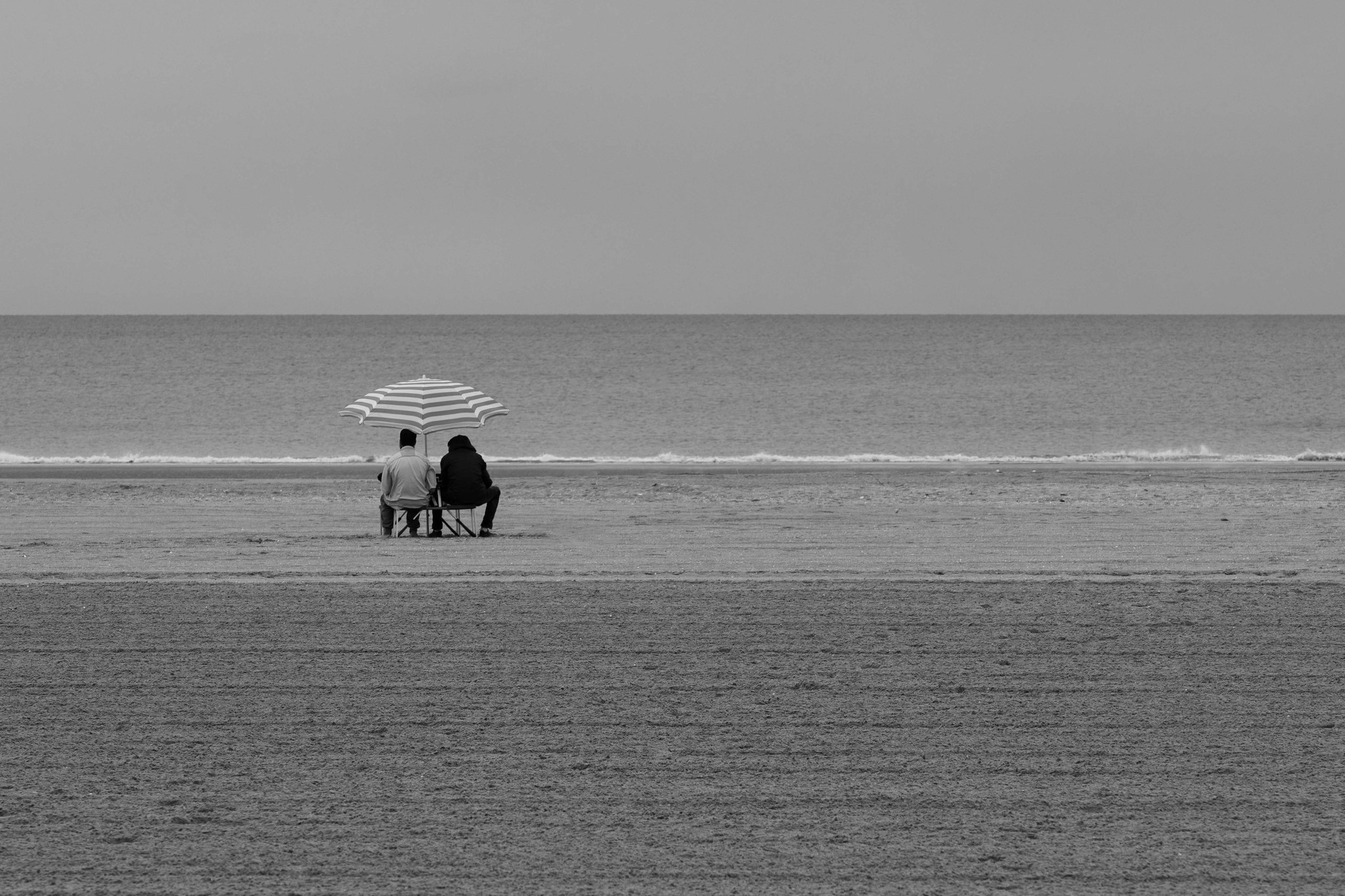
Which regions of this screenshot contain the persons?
[429,434,501,537]
[380,429,437,537]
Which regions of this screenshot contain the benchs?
[391,504,478,539]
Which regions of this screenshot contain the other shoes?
[477,528,497,537]
[429,529,442,537]
[410,532,418,538]
[384,530,392,537]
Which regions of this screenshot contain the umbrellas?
[337,375,510,536]
